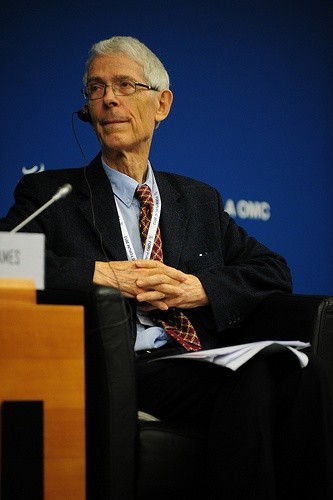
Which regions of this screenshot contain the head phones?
[77,104,93,126]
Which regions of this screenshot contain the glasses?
[81,80,158,100]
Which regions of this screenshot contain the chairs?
[37,292,333,500]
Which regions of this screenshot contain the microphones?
[10,183,74,233]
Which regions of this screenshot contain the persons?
[1,35,333,500]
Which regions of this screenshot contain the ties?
[135,184,201,351]
